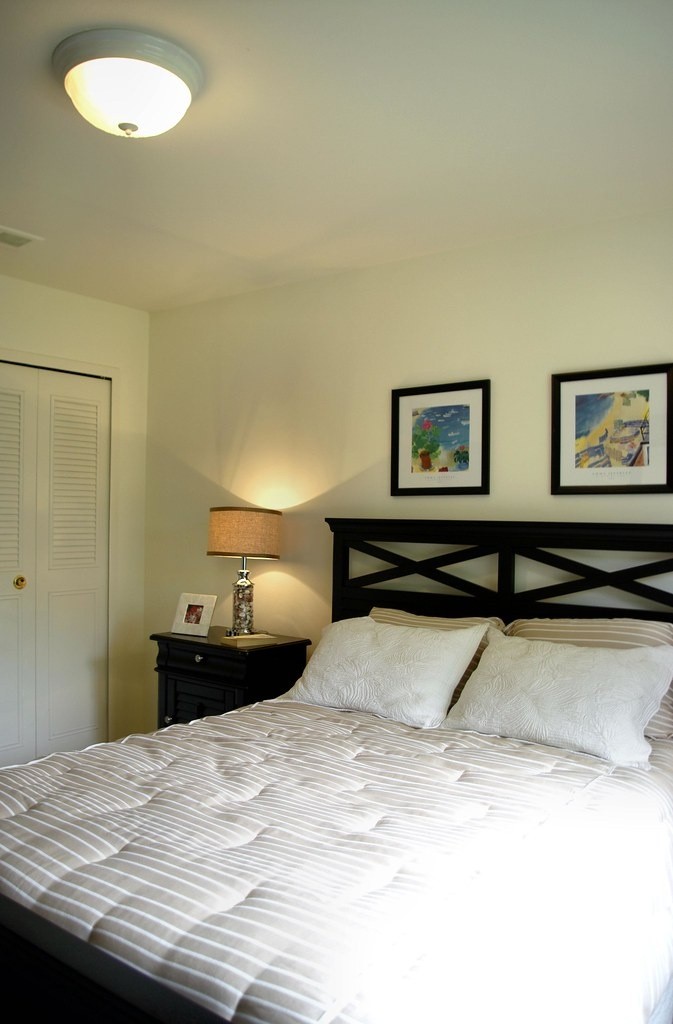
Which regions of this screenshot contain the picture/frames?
[172,589,217,638]
[551,363,673,495]
[391,378,489,495]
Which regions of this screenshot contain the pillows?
[263,607,673,770]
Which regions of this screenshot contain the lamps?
[50,27,202,138]
[207,506,278,637]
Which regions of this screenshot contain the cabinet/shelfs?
[0,355,112,767]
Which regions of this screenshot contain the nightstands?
[149,622,312,729]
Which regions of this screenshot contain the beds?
[0,698,672,1023]
